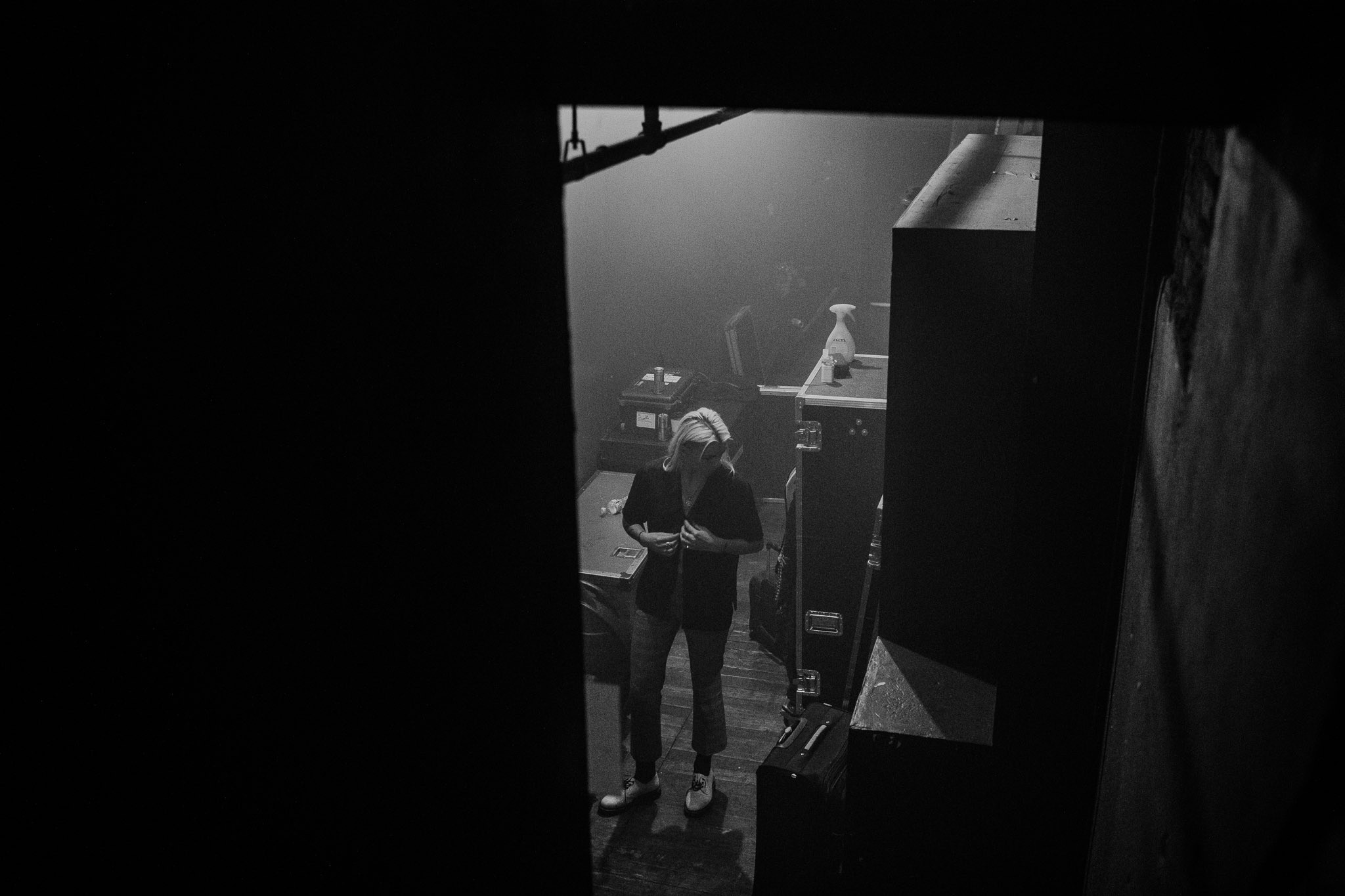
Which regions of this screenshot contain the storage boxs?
[790,353,888,717]
[618,366,702,438]
[578,469,649,676]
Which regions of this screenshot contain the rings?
[685,540,688,545]
[666,542,670,549]
[687,545,689,548]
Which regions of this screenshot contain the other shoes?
[686,767,713,811]
[600,772,661,813]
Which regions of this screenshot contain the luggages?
[752,702,851,896]
[749,541,782,653]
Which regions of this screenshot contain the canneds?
[654,367,664,394]
[658,413,669,441]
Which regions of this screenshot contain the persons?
[598,406,764,811]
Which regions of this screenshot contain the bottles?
[825,356,835,366]
[820,349,830,363]
[600,496,629,515]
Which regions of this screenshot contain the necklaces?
[685,476,705,506]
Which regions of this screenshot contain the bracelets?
[638,531,646,547]
